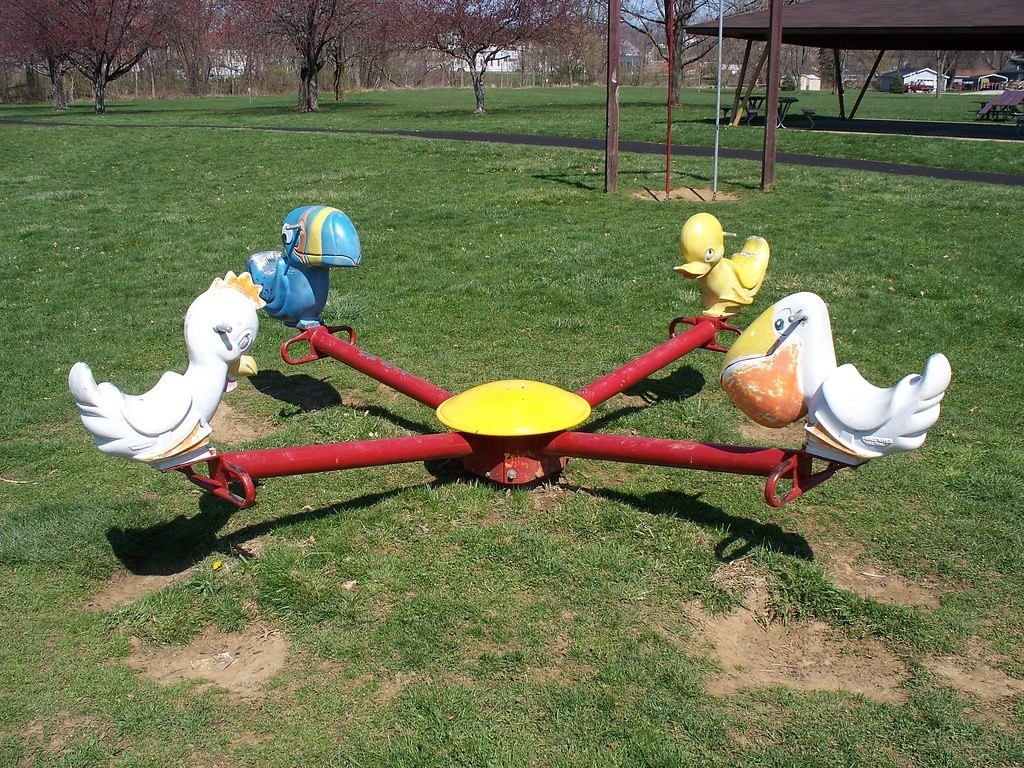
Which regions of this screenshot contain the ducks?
[673,213,769,317]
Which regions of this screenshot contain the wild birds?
[720,291,951,460]
[247,205,362,330]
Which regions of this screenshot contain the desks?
[740,96,799,128]
[990,90,1024,123]
[970,101,1024,122]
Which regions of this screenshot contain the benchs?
[801,108,815,129]
[721,107,759,126]
[967,110,1023,121]
[979,99,996,114]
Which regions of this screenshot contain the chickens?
[68,271,267,463]
